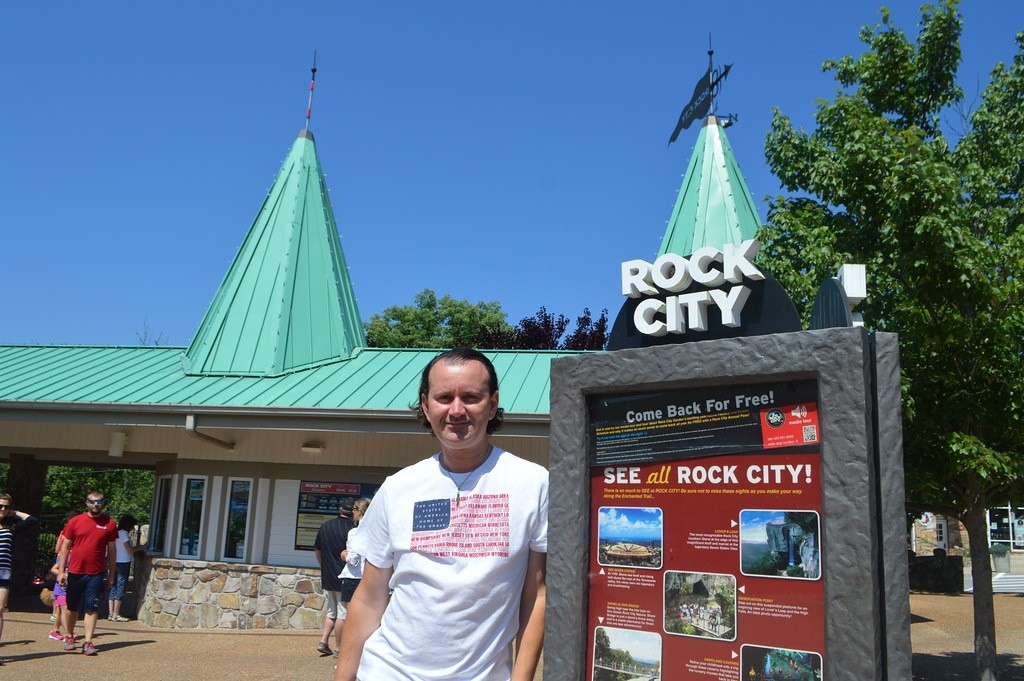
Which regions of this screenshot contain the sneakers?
[48,629,64,641]
[63,635,76,651]
[50,615,56,623]
[81,642,97,655]
[64,632,78,639]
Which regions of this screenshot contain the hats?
[117,514,137,531]
[337,498,355,511]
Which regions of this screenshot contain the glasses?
[87,499,104,504]
[0,505,10,510]
[352,507,357,509]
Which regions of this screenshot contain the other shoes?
[108,615,130,622]
[317,642,333,655]
[334,650,339,658]
[1,661,4,666]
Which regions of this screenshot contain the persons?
[314,496,358,658]
[49,528,78,641]
[749,659,801,680]
[108,513,148,622]
[337,497,371,602]
[677,602,720,631]
[0,494,39,641]
[599,657,645,675]
[56,490,119,655]
[334,347,548,681]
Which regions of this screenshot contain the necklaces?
[442,446,491,507]
